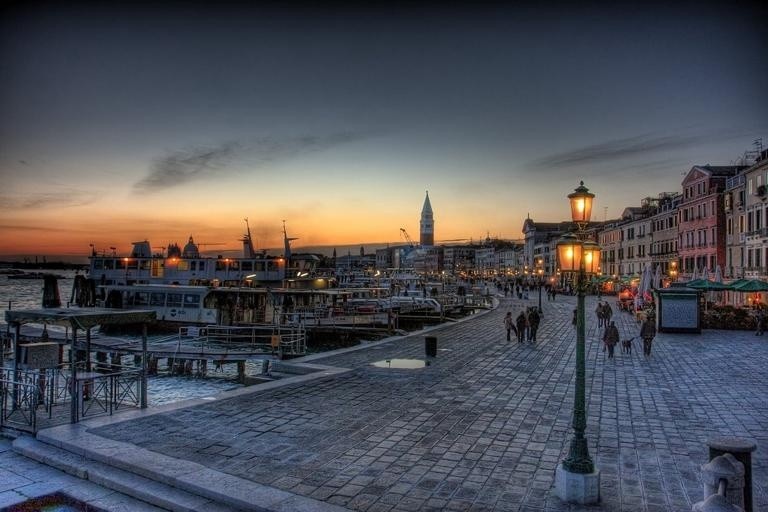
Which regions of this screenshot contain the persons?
[602,320,620,358]
[751,309,763,336]
[498,274,557,302]
[638,316,655,356]
[595,302,603,328]
[602,301,613,328]
[503,311,517,340]
[527,305,540,342]
[515,311,526,343]
[524,307,531,341]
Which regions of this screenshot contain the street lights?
[557,179,602,475]
[537,256,544,314]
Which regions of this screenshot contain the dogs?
[621,337,635,355]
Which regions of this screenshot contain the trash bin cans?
[425,336,437,356]
[524,288,528,299]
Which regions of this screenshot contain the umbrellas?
[632,261,768,310]
